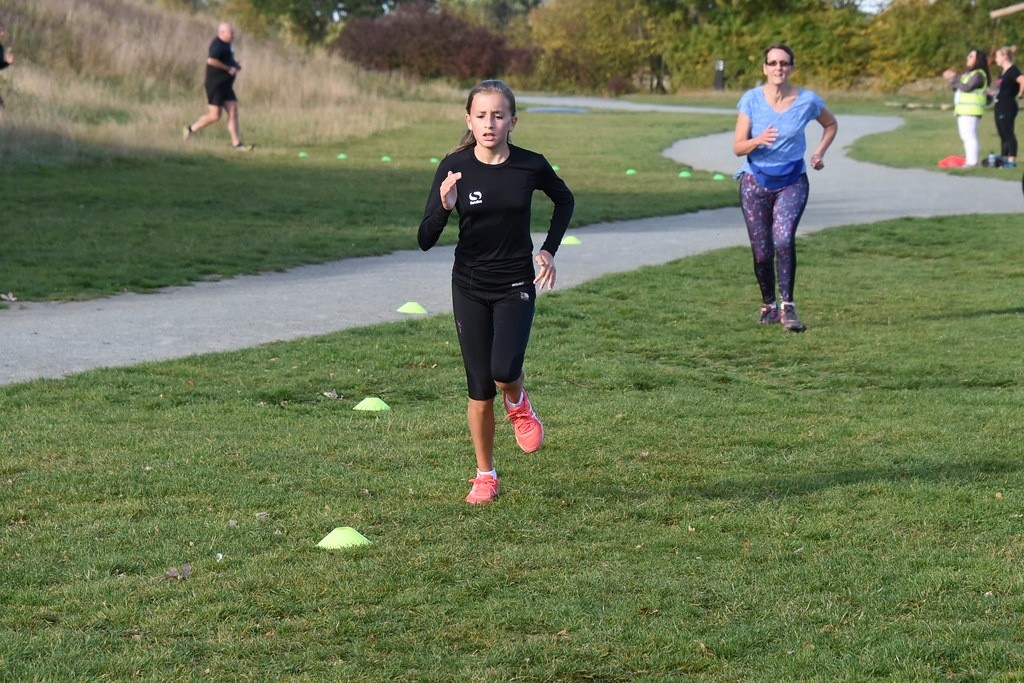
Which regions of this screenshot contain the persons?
[731,45,837,334]
[180,21,254,152]
[0,22,14,108]
[941,45,1024,171]
[418,77,574,502]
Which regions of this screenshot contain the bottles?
[988,152,995,168]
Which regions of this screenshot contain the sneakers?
[464,473,500,505]
[756,302,780,325]
[503,388,544,454]
[780,303,802,331]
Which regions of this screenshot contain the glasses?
[764,61,793,67]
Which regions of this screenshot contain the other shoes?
[182,126,192,145]
[231,144,255,152]
[1001,162,1017,168]
[996,154,1007,164]
